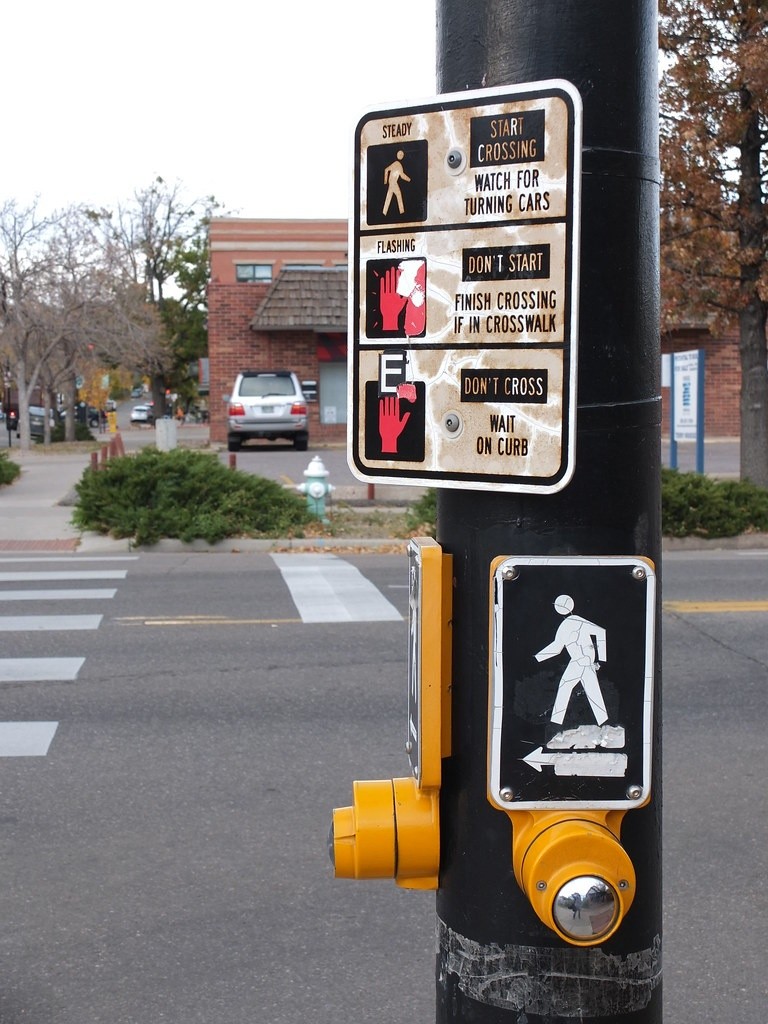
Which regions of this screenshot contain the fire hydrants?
[296,454,337,525]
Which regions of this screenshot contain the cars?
[88,399,116,428]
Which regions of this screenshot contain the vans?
[26,402,63,441]
[129,405,153,424]
[0,402,4,420]
[226,369,310,452]
[130,388,143,398]
